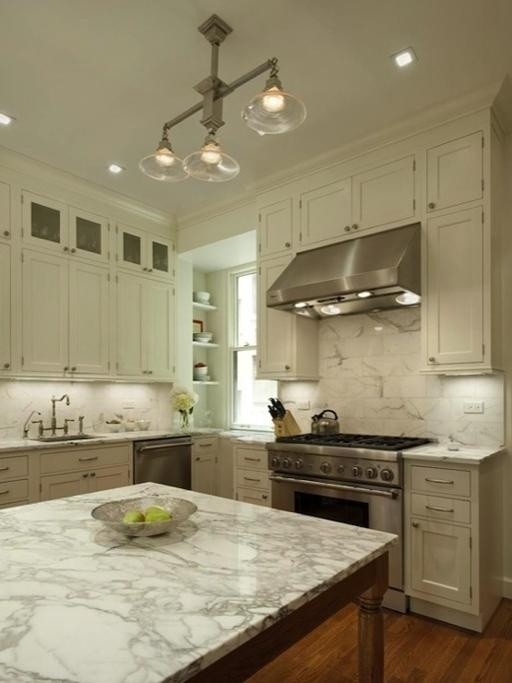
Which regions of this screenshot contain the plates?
[91,496,197,537]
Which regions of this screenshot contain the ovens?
[270,471,403,592]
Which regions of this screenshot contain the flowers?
[170,384,195,414]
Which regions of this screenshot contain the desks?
[1,481,400,682]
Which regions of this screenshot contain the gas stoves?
[264,431,431,487]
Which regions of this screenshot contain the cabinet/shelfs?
[34,433,132,501]
[191,431,221,496]
[297,154,421,252]
[256,197,320,382]
[422,129,512,374]
[115,193,177,381]
[0,441,35,508]
[401,443,504,632]
[193,269,223,383]
[20,155,114,381]
[230,440,276,508]
[0,149,18,381]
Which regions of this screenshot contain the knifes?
[268,397,284,419]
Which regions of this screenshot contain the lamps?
[139,13,306,184]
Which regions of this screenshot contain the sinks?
[32,433,106,444]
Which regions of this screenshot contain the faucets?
[32,395,75,438]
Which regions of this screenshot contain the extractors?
[266,222,421,321]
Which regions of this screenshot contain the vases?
[180,412,194,434]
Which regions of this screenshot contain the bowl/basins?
[195,367,208,377]
[106,421,121,432]
[192,332,213,342]
[124,422,135,431]
[195,292,210,302]
[137,420,151,431]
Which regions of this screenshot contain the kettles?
[311,409,339,436]
